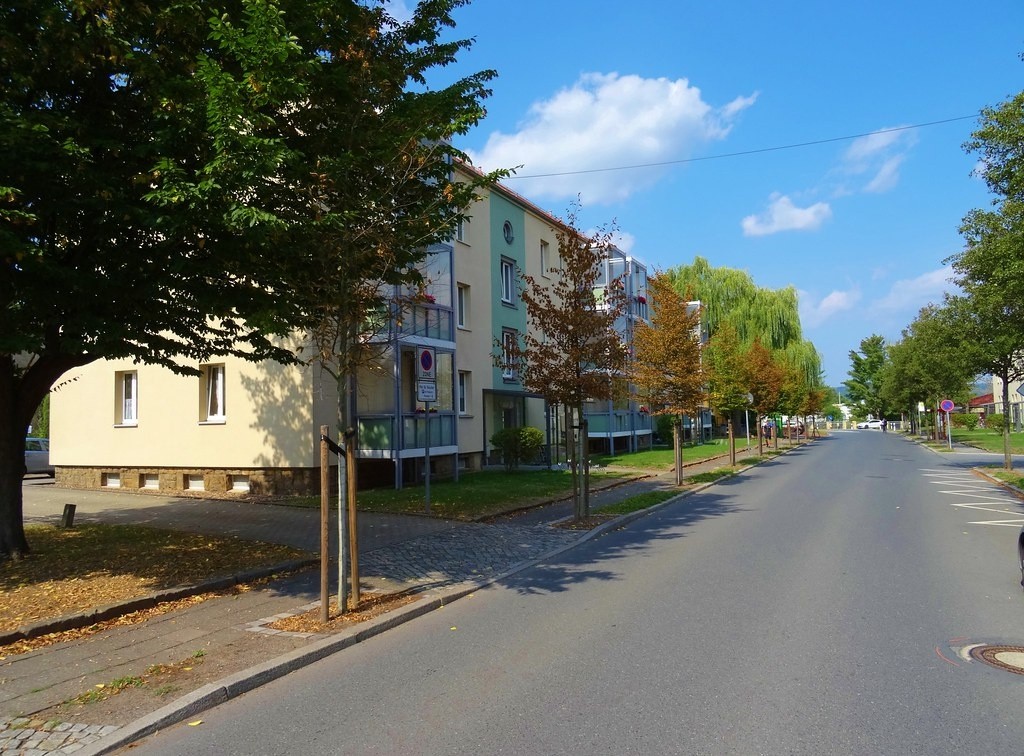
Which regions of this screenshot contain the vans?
[24,438,55,478]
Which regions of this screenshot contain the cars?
[782,421,805,435]
[856,419,882,429]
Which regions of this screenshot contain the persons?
[880,418,886,432]
[763,419,773,448]
